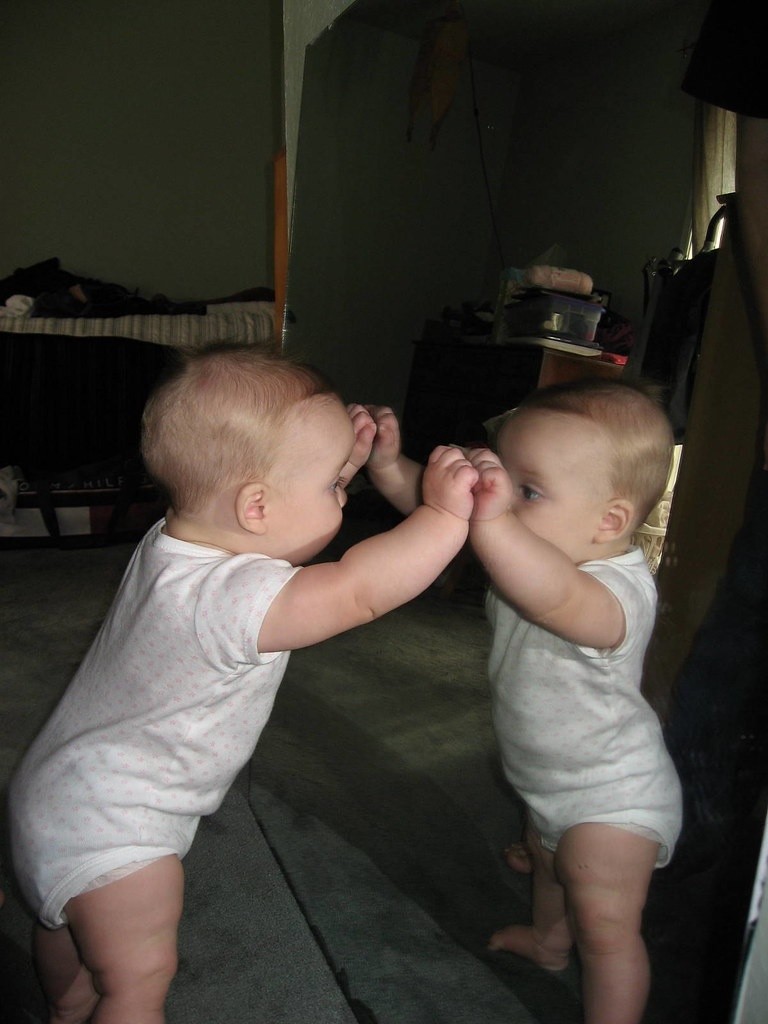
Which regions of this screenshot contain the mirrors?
[247,2,768,1024]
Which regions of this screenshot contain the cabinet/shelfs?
[404,318,629,467]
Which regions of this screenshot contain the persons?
[6,344,475,1024]
[368,374,685,1023]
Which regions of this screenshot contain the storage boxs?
[504,295,605,341]
